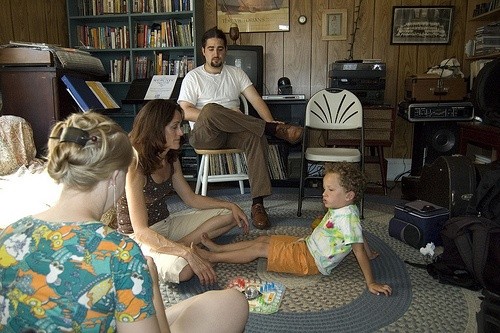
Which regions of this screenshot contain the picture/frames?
[216,0,290,35]
[322,9,348,40]
[390,6,454,46]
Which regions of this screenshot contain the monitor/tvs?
[226,45,263,97]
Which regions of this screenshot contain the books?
[132,0,193,13]
[135,17,194,48]
[210,151,248,175]
[77,25,129,49]
[110,56,131,82]
[267,145,289,179]
[135,51,195,78]
[78,0,128,16]
[474,24,500,56]
[62,74,119,111]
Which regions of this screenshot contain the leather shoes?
[251,204,271,229]
[274,123,304,144]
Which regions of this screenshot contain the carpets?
[158,194,484,333]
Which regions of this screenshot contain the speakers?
[409,121,462,175]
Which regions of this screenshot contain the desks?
[411,119,500,177]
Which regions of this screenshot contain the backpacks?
[442,215,500,288]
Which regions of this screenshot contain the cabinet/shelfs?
[324,106,395,195]
[262,101,307,188]
[65,0,203,134]
[464,0,500,62]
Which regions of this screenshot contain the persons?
[191,161,392,297]
[0,113,249,333]
[397,22,446,38]
[100,99,250,287]
[177,29,303,229]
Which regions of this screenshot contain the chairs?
[188,93,249,196]
[298,88,365,219]
